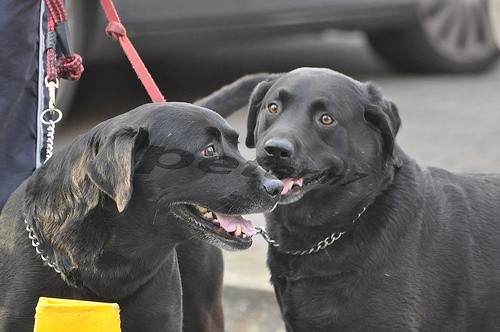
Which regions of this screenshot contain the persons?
[0,0,65,207]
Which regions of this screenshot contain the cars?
[56,0,498,117]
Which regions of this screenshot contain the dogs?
[245,66,500,331]
[0,73,284,332]
